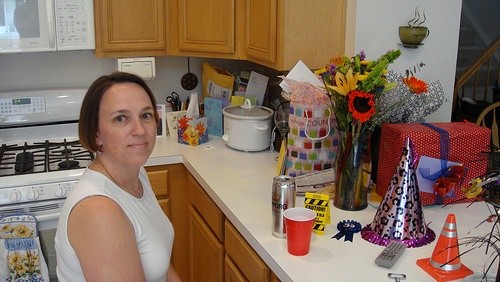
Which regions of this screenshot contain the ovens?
[0,207,68,278]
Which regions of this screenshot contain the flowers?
[434,142,500,282]
[314,48,429,205]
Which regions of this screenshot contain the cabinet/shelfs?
[91,0,347,71]
[143,163,284,282]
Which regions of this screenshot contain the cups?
[399,25,429,48]
[283,207,316,256]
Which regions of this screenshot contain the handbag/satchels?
[284,101,340,196]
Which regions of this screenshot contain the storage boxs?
[376,120,491,205]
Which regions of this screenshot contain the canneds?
[271,175,297,239]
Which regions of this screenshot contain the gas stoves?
[0,89,97,205]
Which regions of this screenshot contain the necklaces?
[97,158,143,198]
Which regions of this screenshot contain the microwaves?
[0,0,96,54]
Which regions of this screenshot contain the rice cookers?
[222,99,274,152]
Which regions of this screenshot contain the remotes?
[375,241,406,268]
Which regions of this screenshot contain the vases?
[333,129,372,211]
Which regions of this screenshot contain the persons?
[53,71,174,282]
